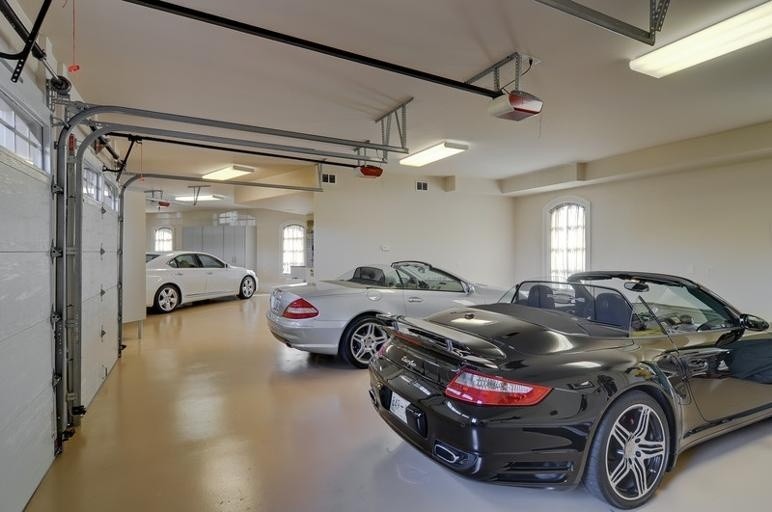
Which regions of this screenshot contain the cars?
[146,250,259,313]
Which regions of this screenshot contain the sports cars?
[266,259,529,369]
[367,271,771,511]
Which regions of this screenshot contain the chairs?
[354,266,386,284]
[527,282,555,310]
[590,290,634,330]
